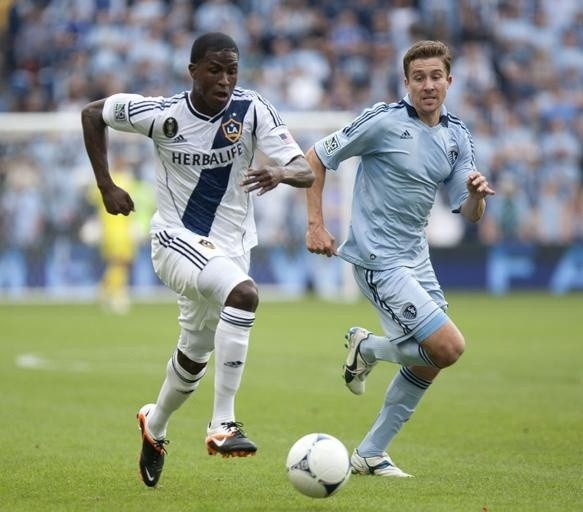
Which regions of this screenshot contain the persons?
[80,32,315,487]
[304,41,496,485]
[1,0,582,295]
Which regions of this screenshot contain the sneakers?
[205,420,258,458]
[343,327,376,396]
[136,404,166,488]
[351,448,415,478]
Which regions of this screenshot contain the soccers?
[287,432,352,499]
